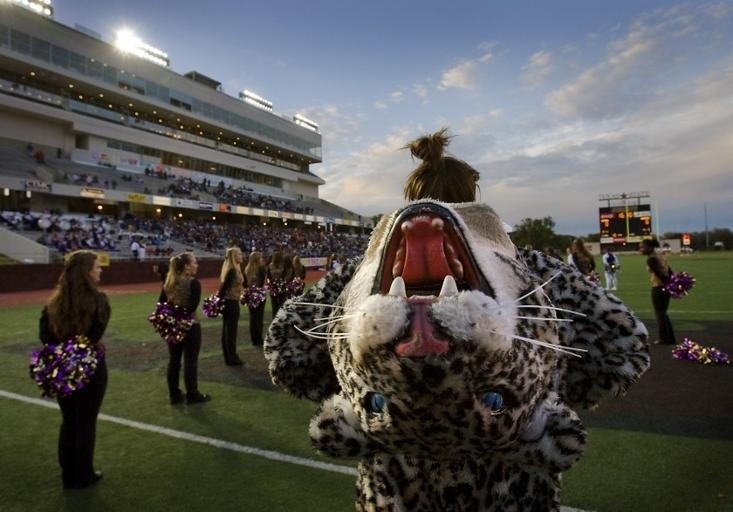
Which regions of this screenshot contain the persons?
[241,251,267,346]
[156,251,211,406]
[521,236,602,287]
[265,251,288,320]
[601,246,622,291]
[281,254,296,302]
[259,125,653,511]
[291,254,307,295]
[216,246,246,366]
[640,238,677,346]
[36,248,112,490]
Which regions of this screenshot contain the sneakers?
[653,337,676,345]
[225,358,247,366]
[170,389,211,406]
[605,286,618,291]
[64,469,103,489]
